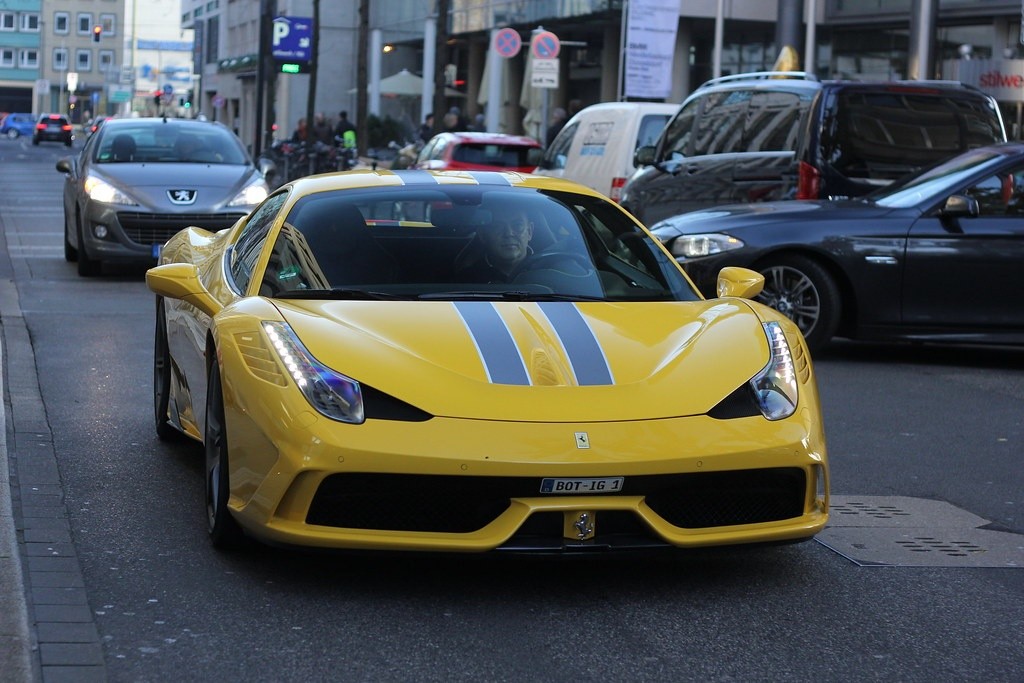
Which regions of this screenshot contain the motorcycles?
[262,138,356,184]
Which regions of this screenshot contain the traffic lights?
[154,89,161,106]
[179,97,191,107]
[94,26,100,44]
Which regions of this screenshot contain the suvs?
[619,71,1014,230]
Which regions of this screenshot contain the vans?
[530,101,693,204]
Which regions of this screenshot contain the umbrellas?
[477,29,512,133]
[520,26,564,144]
[345,70,470,122]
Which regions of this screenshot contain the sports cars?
[146,169,829,557]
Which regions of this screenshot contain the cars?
[649,143,1024,361]
[56,117,278,277]
[0,113,75,147]
[407,132,547,174]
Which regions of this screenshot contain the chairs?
[111,134,137,160]
[296,203,371,284]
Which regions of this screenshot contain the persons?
[434,198,573,295]
[292,109,416,176]
[546,100,583,149]
[419,107,487,144]
